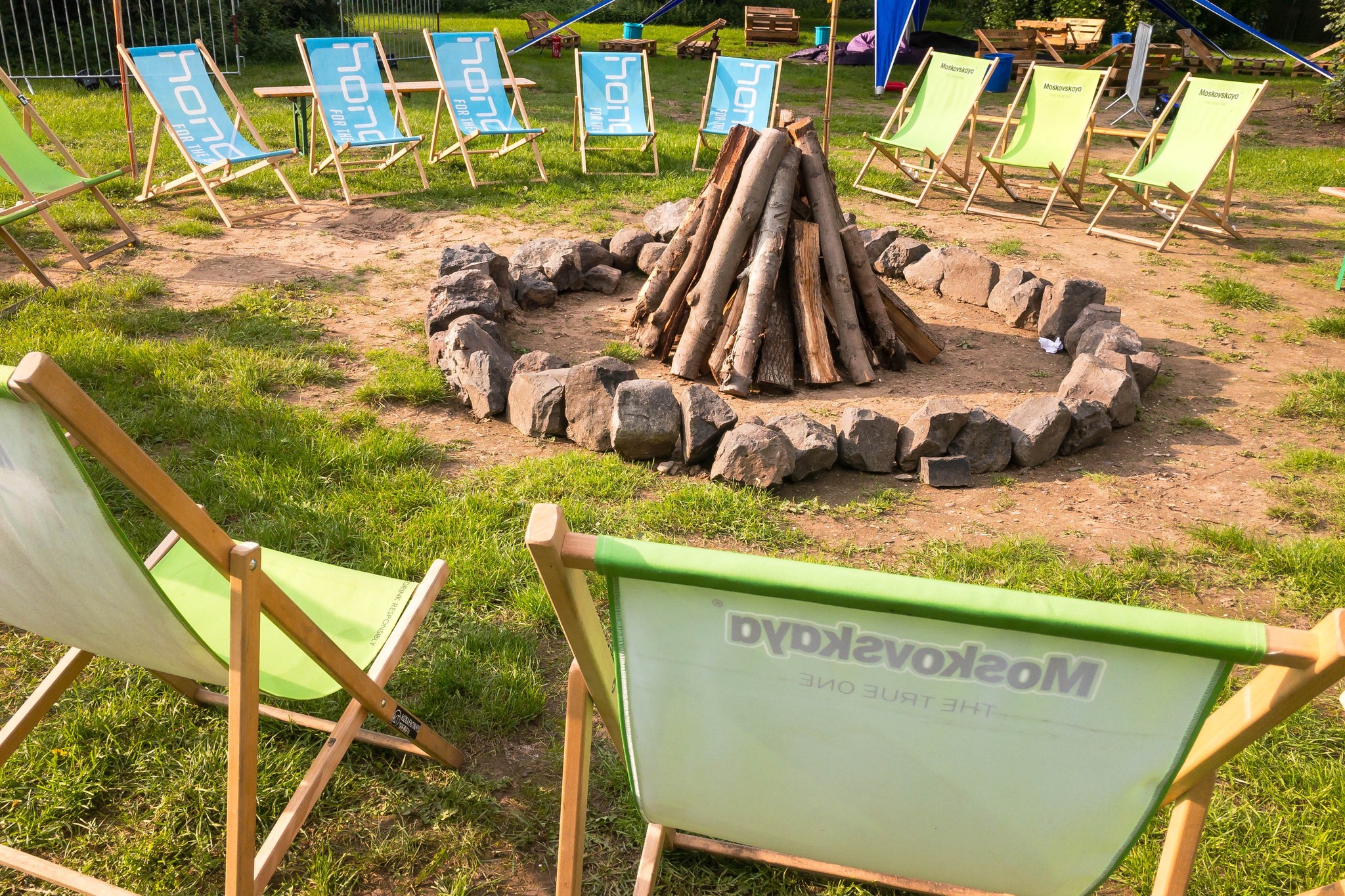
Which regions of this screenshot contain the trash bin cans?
[1111,32,1132,54]
[815,27,830,46]
[623,22,644,39]
[983,53,1015,92]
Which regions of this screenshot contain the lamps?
[100,69,122,90]
[1156,94,1174,104]
[377,54,398,70]
[74,69,102,92]
[1152,96,1180,124]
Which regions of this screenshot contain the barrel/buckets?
[623,22,644,40]
[983,53,1016,93]
[1112,32,1132,55]
[815,26,831,47]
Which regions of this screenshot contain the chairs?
[0,6,1345,896]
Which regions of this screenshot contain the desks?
[1231,57,1285,77]
[598,39,657,57]
[252,76,534,156]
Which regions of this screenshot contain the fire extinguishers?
[540,31,563,58]
